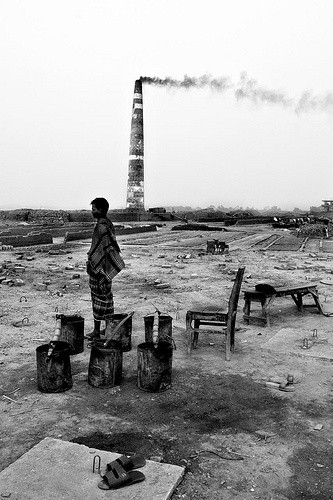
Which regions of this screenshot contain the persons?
[84,198,125,340]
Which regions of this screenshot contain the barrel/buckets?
[88,339,122,389]
[144,316,172,342]
[105,314,132,352]
[56,314,84,355]
[137,343,173,392]
[36,343,73,393]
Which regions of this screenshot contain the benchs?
[243,284,323,329]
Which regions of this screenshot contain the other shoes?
[86,331,100,338]
[100,329,105,335]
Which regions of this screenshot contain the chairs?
[187,265,246,362]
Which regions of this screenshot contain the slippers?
[97,466,146,491]
[99,453,146,478]
